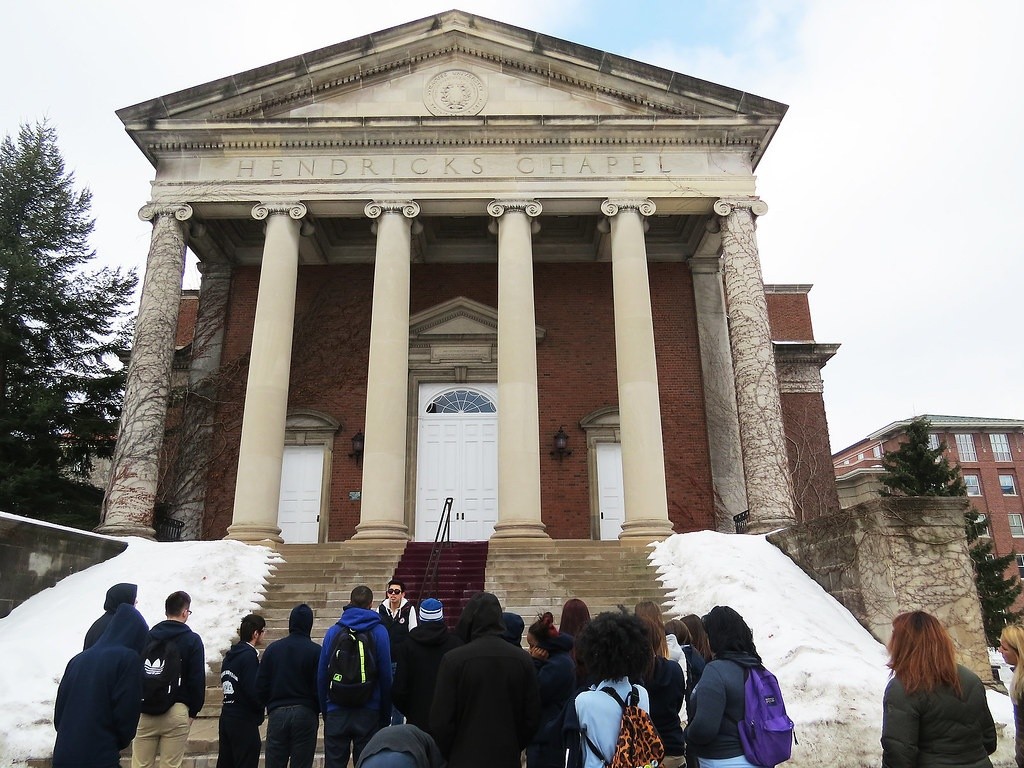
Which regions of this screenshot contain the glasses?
[184,610,192,615]
[387,589,402,595]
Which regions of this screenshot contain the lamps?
[347,429,364,465]
[550,427,572,464]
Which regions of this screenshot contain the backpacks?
[326,622,378,694]
[708,658,799,767]
[582,687,664,768]
[141,632,184,701]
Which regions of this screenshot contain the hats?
[419,598,443,622]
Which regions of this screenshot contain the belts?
[276,705,303,709]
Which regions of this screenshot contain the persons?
[318,579,760,768]
[83,582,137,649]
[51,603,149,768]
[880,611,997,768]
[216,604,322,768]
[998,625,1024,768]
[131,591,206,768]
